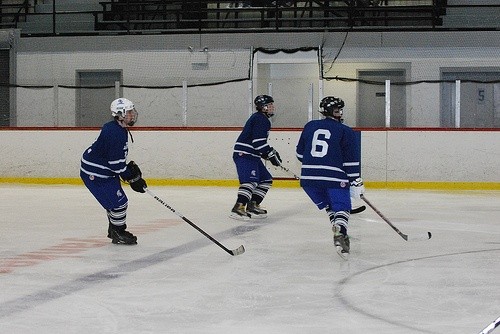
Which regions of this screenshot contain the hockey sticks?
[141,186,246,256]
[279,163,366,214]
[360,193,432,242]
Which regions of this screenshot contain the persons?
[296,96,365,261]
[227,95,282,222]
[79,97,147,245]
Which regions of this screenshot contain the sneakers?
[107,218,113,239]
[246,200,268,219]
[333,225,350,261]
[111,223,138,245]
[228,201,252,221]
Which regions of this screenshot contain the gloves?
[127,161,142,177]
[261,153,269,160]
[125,167,147,193]
[350,177,364,198]
[267,147,282,167]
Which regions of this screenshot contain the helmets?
[111,98,138,126]
[254,94,275,118]
[319,96,345,123]
